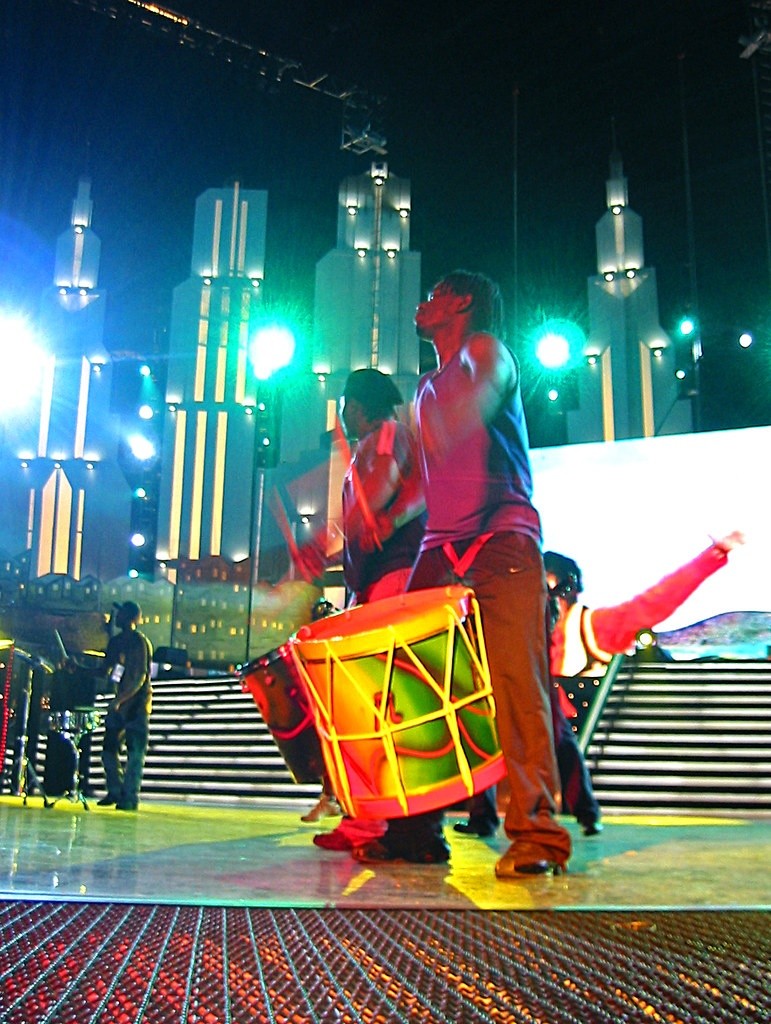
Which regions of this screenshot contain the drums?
[288,585,509,820]
[41,710,101,732]
[237,642,329,785]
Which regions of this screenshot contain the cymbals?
[13,646,54,674]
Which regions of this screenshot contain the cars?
[151,644,196,681]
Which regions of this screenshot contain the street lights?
[235,327,294,663]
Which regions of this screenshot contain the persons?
[298,368,430,853]
[96,602,154,810]
[453,587,603,839]
[541,529,743,721]
[353,272,571,877]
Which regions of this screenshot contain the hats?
[113,600,142,620]
[543,550,584,594]
[346,368,404,408]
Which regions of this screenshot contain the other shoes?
[352,818,406,863]
[301,793,341,822]
[97,792,120,805]
[454,820,491,835]
[403,816,450,863]
[495,840,568,879]
[116,804,125,809]
[578,806,604,836]
[314,815,379,851]
